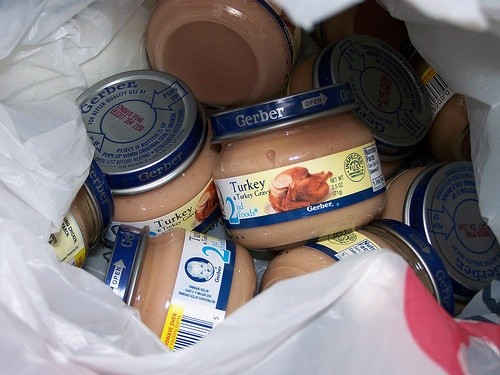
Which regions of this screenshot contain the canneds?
[45,0,499,353]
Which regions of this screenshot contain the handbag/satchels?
[0,0,499,375]
